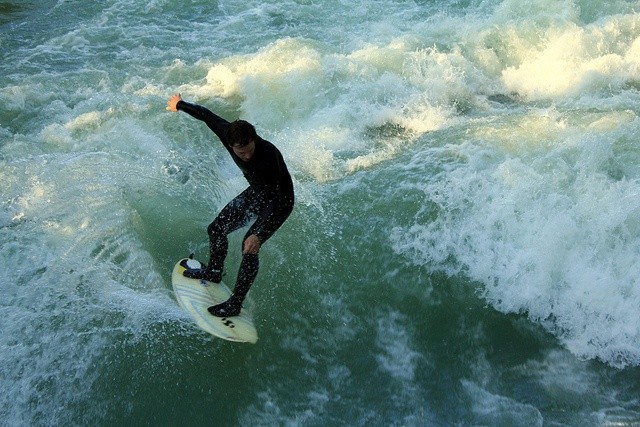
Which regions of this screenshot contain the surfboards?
[172,255,259,344]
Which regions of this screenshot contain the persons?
[165,92,294,317]
